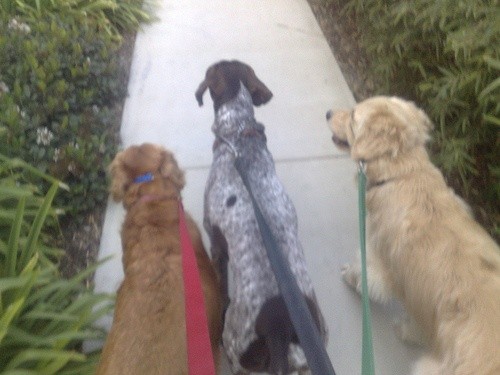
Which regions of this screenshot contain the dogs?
[94,144,222,375]
[194,60,332,375]
[325,96,500,375]
